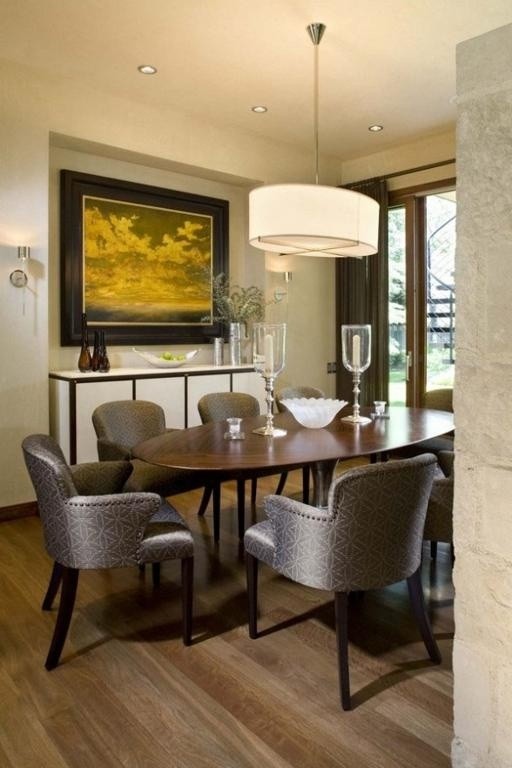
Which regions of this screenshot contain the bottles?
[214,338,225,366]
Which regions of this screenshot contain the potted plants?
[199,268,262,366]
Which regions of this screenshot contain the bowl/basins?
[278,397,350,430]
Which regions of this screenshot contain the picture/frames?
[59,167,230,347]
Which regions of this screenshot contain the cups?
[374,401,387,416]
[228,419,242,435]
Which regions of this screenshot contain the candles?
[264,335,273,373]
[353,334,361,367]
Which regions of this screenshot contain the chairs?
[198,392,268,516]
[421,388,453,411]
[244,453,444,711]
[91,401,221,585]
[20,433,196,672]
[273,385,329,507]
[423,449,456,558]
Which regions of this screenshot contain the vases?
[79,312,110,373]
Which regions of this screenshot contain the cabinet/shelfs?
[49,363,272,465]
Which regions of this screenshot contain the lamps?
[9,246,32,316]
[264,252,297,300]
[247,21,380,260]
